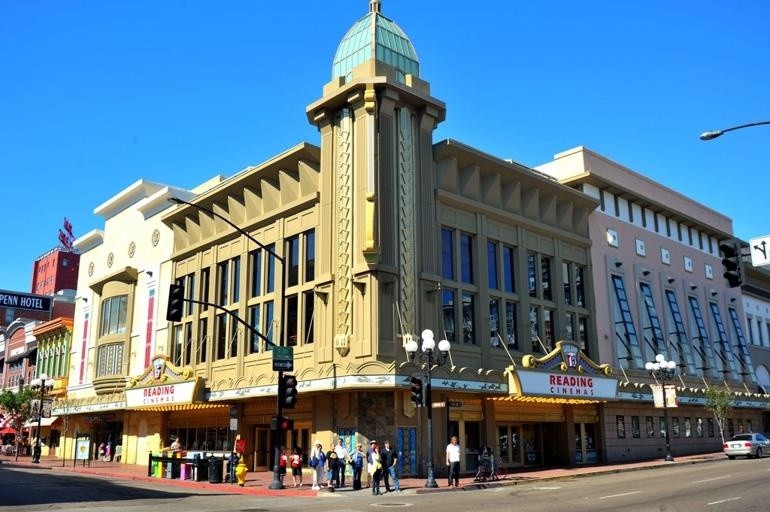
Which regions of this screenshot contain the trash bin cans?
[208,458,223,483]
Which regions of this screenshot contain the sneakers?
[448,485,465,489]
[282,484,403,495]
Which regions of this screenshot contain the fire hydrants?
[234,454,249,487]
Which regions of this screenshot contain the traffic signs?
[272,346,294,372]
[430,401,445,408]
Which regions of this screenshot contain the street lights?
[168,196,286,490]
[406,328,452,491]
[28,372,55,463]
[699,120,770,143]
[643,353,678,460]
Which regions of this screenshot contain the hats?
[370,439,377,444]
[355,443,362,448]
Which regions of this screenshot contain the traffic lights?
[282,374,298,409]
[409,375,423,406]
[165,283,185,323]
[281,416,294,432]
[717,240,742,285]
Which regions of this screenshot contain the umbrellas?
[0,427,17,444]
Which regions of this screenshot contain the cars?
[721,432,770,459]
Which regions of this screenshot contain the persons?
[171,434,182,449]
[446,436,461,487]
[289,444,304,487]
[308,436,400,495]
[0,435,29,456]
[279,447,288,487]
[473,445,490,481]
[31,434,41,459]
